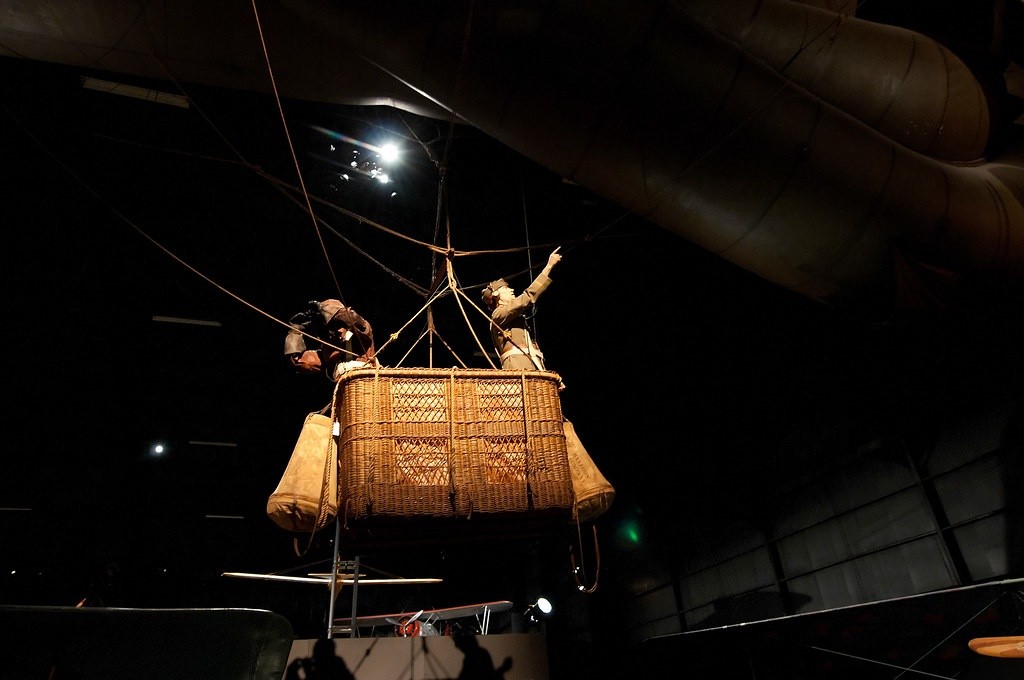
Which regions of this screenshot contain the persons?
[482,246,562,369]
[283,299,375,382]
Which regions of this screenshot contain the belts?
[500,347,544,365]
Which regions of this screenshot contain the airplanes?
[333,600,513,639]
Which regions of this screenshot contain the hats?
[481,278,509,306]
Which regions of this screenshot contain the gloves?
[305,301,323,317]
[289,312,313,329]
